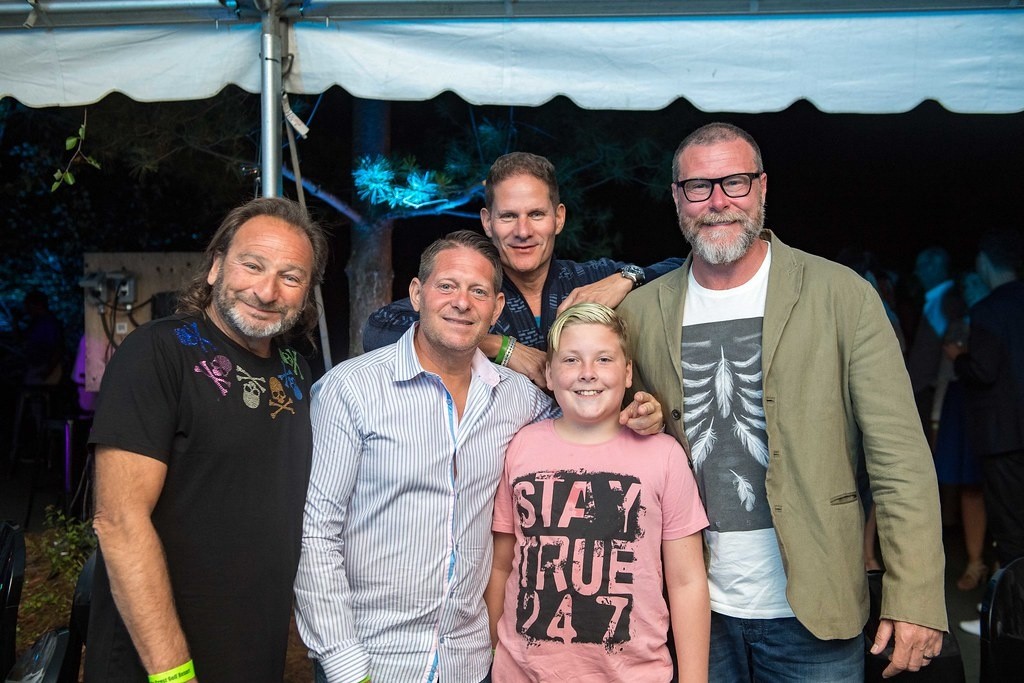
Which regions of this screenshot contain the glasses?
[678,172,760,203]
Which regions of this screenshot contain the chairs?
[979,556,1024,683]
[55,547,96,683]
[864,570,967,683]
[0,519,29,670]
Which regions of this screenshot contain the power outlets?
[106,270,137,304]
[78,270,109,306]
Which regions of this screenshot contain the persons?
[484,303,710,683]
[295,231,663,683]
[616,123,949,683]
[86,199,329,683]
[823,227,1024,586]
[362,154,688,388]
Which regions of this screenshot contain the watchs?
[621,264,645,290]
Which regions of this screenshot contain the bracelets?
[360,676,370,683]
[495,335,517,366]
[148,660,195,683]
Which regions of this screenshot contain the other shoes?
[958,564,987,590]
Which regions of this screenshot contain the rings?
[923,654,933,660]
